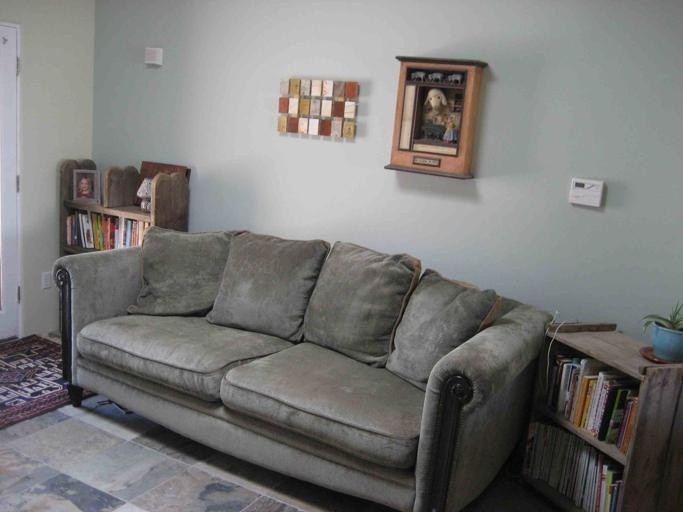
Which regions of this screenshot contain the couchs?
[50,224,549,509]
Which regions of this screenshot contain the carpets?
[0,332,95,431]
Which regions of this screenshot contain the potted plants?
[642,307,681,360]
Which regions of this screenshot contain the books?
[521,341,640,512]
[66,209,153,251]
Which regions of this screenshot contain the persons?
[443,115,456,144]
[76,176,94,198]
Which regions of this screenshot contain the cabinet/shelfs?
[520,325,681,511]
[58,158,190,257]
